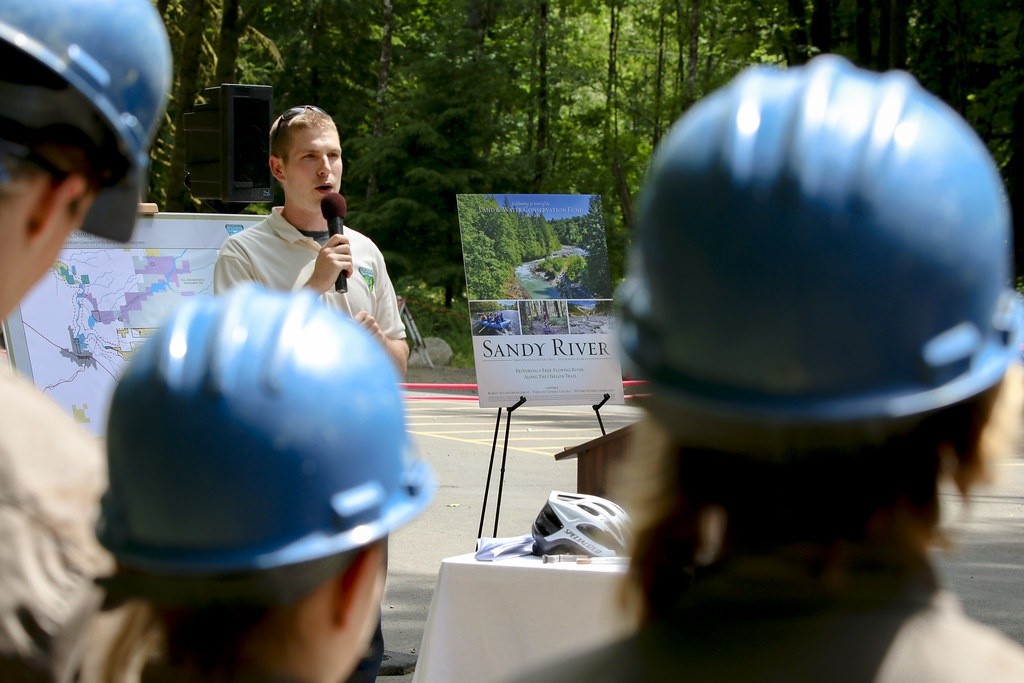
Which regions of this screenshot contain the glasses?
[274,104,329,155]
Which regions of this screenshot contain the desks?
[411,551,642,683]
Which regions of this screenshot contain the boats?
[476,315,512,330]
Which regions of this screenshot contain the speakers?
[180,83,274,215]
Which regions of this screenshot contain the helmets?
[530,490,635,559]
[96,280,439,574]
[610,52,1024,424]
[0,1,173,244]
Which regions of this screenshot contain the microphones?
[320,192,347,294]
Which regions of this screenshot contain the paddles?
[474,322,481,328]
[500,325,509,335]
[478,322,490,333]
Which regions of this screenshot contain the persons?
[500,56,1024,683]
[0,0,437,683]
